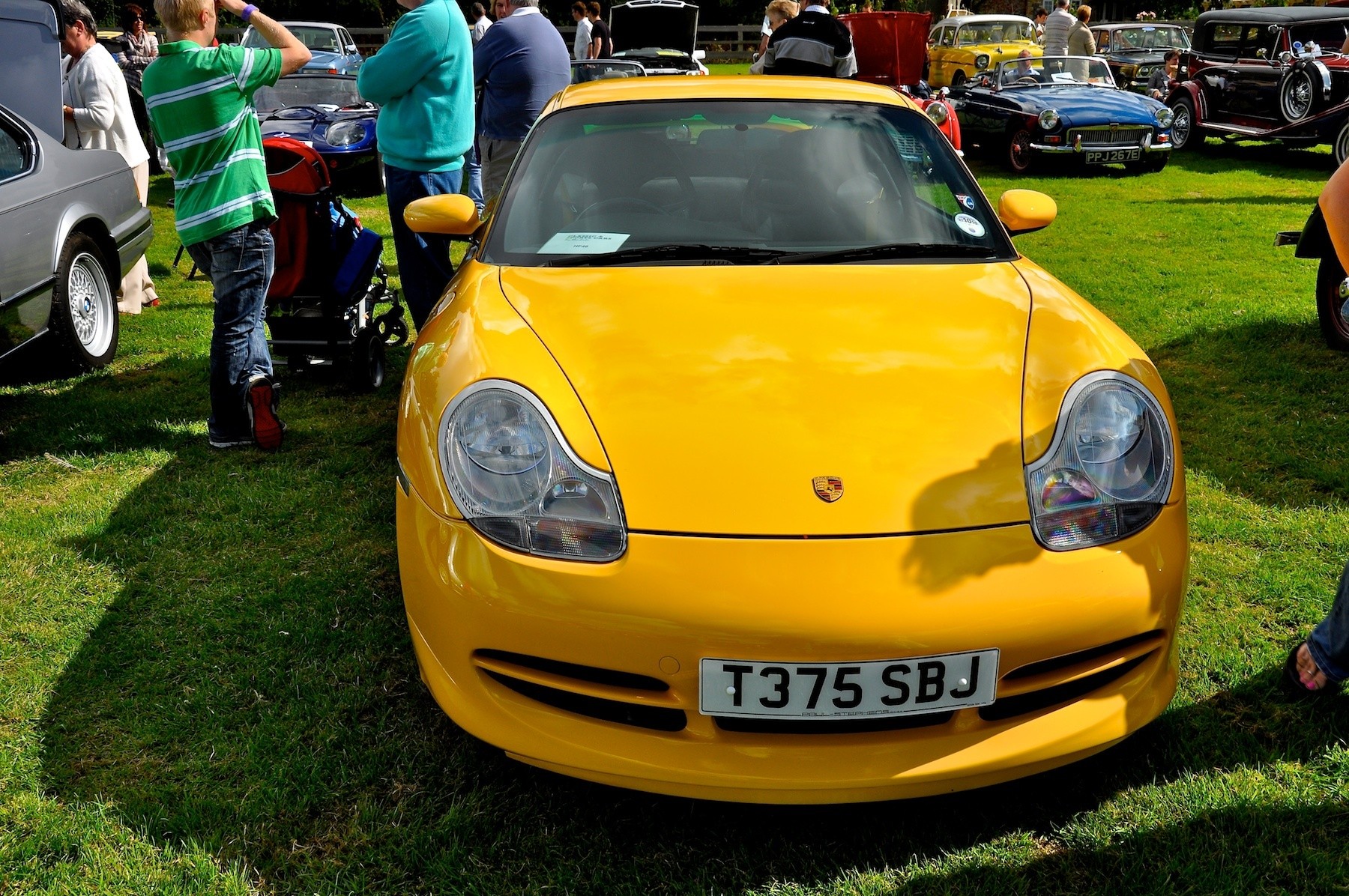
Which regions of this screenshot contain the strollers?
[261,138,409,396]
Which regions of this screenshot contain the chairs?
[308,37,333,49]
[695,128,792,177]
[744,127,904,244]
[538,126,699,223]
[977,30,984,40]
[991,30,1004,43]
[602,71,628,79]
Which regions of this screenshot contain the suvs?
[923,13,1046,95]
[1162,6,1349,168]
[1089,23,1192,96]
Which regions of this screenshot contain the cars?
[395,73,1190,807]
[570,58,647,85]
[609,0,710,76]
[251,50,386,196]
[238,20,365,80]
[1271,157,1349,354]
[62,31,168,177]
[835,11,962,184]
[943,55,1176,177]
[0,1,155,381]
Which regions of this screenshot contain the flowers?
[1136,11,1157,19]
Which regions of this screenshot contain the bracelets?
[241,4,259,21]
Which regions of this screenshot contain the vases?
[1143,16,1154,21]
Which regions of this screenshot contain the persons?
[142,0,312,446]
[48,0,159,314]
[572,2,613,83]
[468,1,571,219]
[1026,0,1096,81]
[1005,50,1040,83]
[1146,50,1180,102]
[1285,560,1349,693]
[357,0,476,334]
[750,0,858,79]
[1099,30,1133,52]
[117,6,159,158]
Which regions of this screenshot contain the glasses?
[133,16,144,21]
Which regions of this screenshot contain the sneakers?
[208,420,289,449]
[241,374,285,452]
